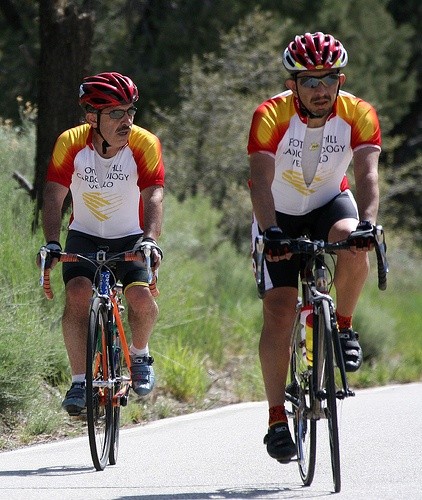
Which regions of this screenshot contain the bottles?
[299,305,313,362]
[305,314,313,366]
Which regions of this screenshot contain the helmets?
[78,72,139,110]
[282,32,348,72]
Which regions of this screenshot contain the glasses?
[95,108,137,119]
[296,72,338,88]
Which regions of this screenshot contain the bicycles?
[40,245,160,470]
[254,225,388,492]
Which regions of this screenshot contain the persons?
[245,32,383,459]
[35,72,166,416]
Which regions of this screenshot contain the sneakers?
[129,355,155,396]
[62,381,87,412]
[334,329,363,372]
[263,422,297,459]
